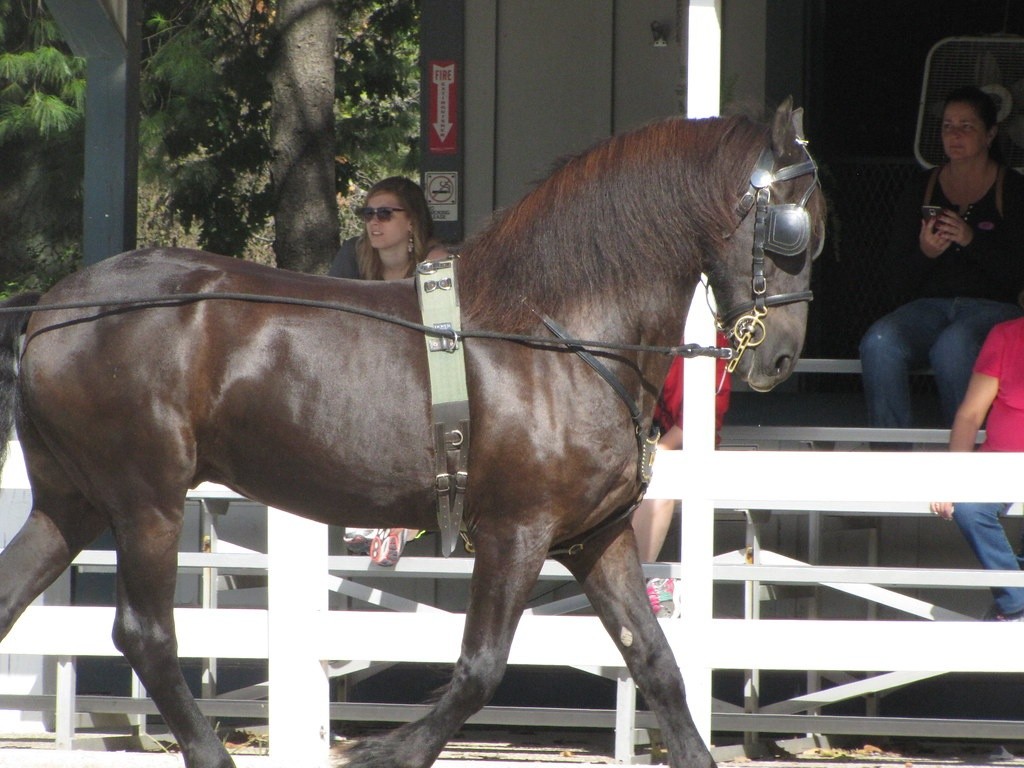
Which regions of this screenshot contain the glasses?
[355,206,406,222]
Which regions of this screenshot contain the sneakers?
[344,526,410,566]
[642,577,680,616]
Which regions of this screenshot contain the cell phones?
[920,205,945,233]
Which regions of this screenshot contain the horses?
[1,94,826,768]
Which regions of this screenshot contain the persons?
[930,315,1024,621]
[859,88,1024,451]
[318,178,451,567]
[628,330,731,583]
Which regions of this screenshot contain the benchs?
[56,357,1024,764]
